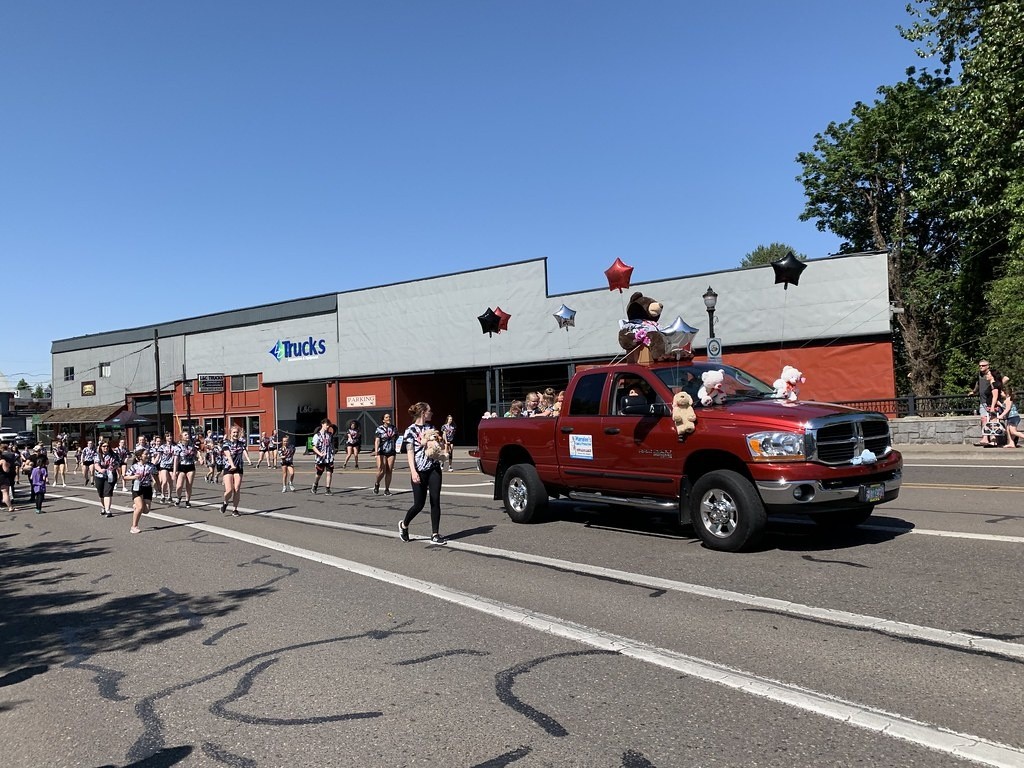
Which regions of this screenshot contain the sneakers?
[440,463,454,472]
[232,510,241,517]
[220,506,226,514]
[113,483,128,492]
[83,480,94,487]
[282,486,287,493]
[100,508,112,517]
[343,463,346,469]
[130,526,140,533]
[310,488,317,494]
[354,465,359,470]
[248,463,260,469]
[51,481,67,488]
[325,489,332,496]
[430,533,447,546]
[290,485,295,491]
[268,466,276,470]
[373,488,379,495]
[159,497,191,508]
[204,475,218,484]
[398,519,409,543]
[384,489,392,497]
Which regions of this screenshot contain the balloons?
[604,257,634,291]
[553,304,576,329]
[770,251,807,286]
[477,307,511,334]
[661,316,699,359]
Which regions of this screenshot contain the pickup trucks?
[467,359,903,551]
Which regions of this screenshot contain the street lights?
[703,285,719,337]
[184,382,195,447]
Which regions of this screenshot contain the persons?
[281,435,297,492]
[399,402,447,545]
[0,426,253,533]
[266,429,282,470]
[440,415,455,472]
[968,359,1024,448]
[504,388,565,417]
[257,431,270,469]
[310,419,335,495]
[343,420,362,469]
[626,384,651,406]
[373,414,399,496]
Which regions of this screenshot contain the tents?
[94,411,167,451]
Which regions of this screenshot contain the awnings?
[34,406,124,425]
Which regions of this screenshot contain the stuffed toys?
[618,293,665,396]
[698,369,726,406]
[850,450,877,466]
[773,366,806,401]
[421,429,449,463]
[482,412,498,420]
[672,392,696,442]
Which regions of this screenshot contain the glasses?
[101,445,109,447]
[977,364,988,368]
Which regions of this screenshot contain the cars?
[0,427,18,444]
[14,430,36,449]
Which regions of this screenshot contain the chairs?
[618,382,648,415]
[682,377,704,401]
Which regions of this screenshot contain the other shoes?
[974,442,1016,448]
